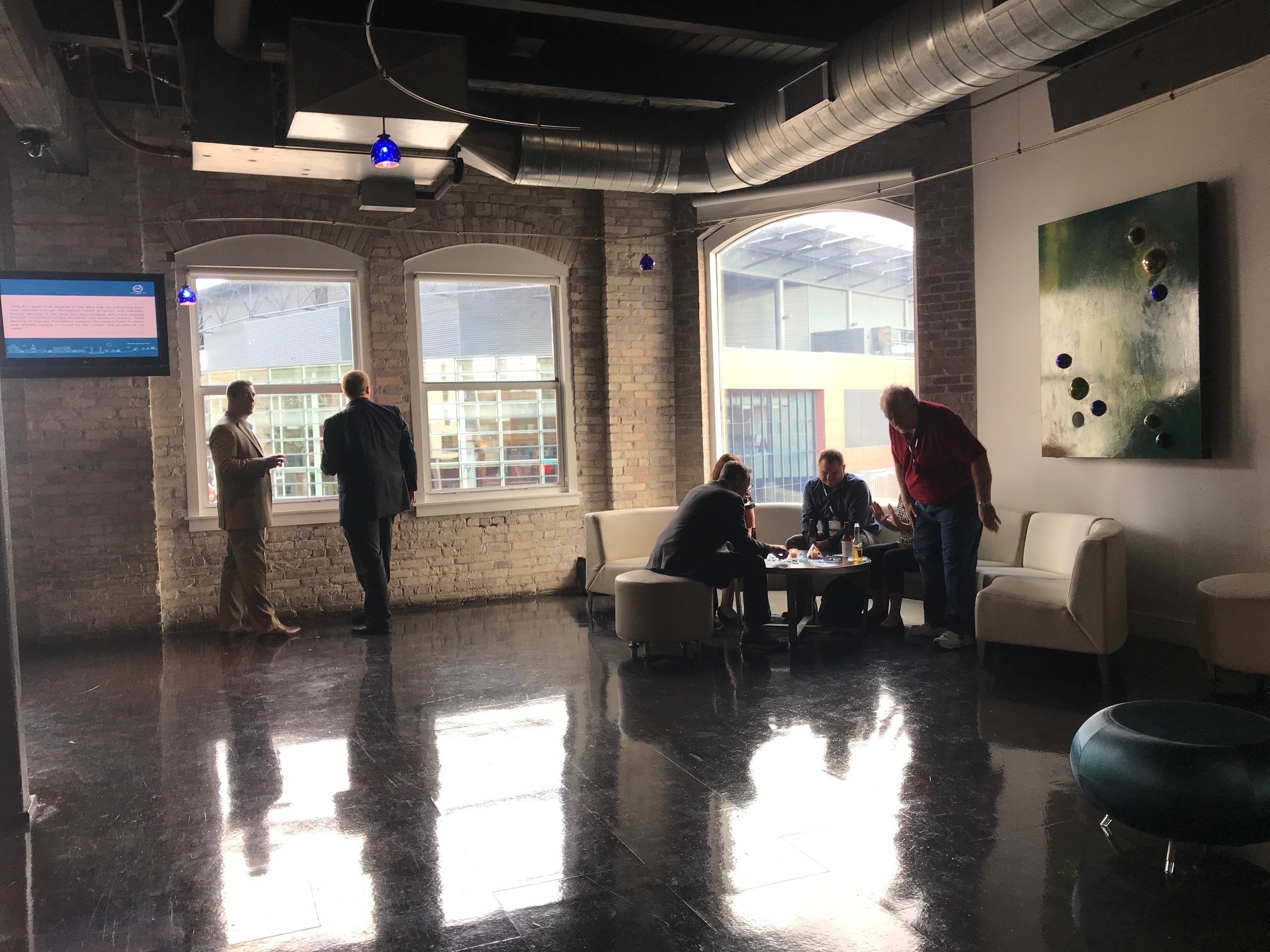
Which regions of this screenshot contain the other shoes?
[874,619,904,633]
[781,612,788,618]
[867,613,886,626]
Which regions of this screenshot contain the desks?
[734,550,872,665]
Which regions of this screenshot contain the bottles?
[852,523,863,562]
[841,522,852,557]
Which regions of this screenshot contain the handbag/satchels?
[819,577,862,626]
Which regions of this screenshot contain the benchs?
[585,502,1130,668]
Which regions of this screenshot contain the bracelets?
[978,500,991,505]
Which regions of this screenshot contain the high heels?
[717,607,736,622]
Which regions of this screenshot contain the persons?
[646,454,789,649]
[209,380,301,641]
[320,369,418,638]
[786,385,1001,652]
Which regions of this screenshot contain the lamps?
[371,70,401,169]
[177,220,196,306]
[640,236,655,272]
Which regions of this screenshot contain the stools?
[1196,574,1270,681]
[1070,699,1270,880]
[614,569,714,674]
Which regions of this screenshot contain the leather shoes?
[352,613,367,623]
[219,626,254,635]
[257,623,301,640]
[352,625,392,634]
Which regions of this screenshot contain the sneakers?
[714,620,725,632]
[934,631,973,650]
[739,630,789,649]
[911,622,949,636]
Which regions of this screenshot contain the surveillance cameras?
[18,128,50,158]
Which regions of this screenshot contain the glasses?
[741,478,753,490]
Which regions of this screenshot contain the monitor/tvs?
[0,270,170,377]
